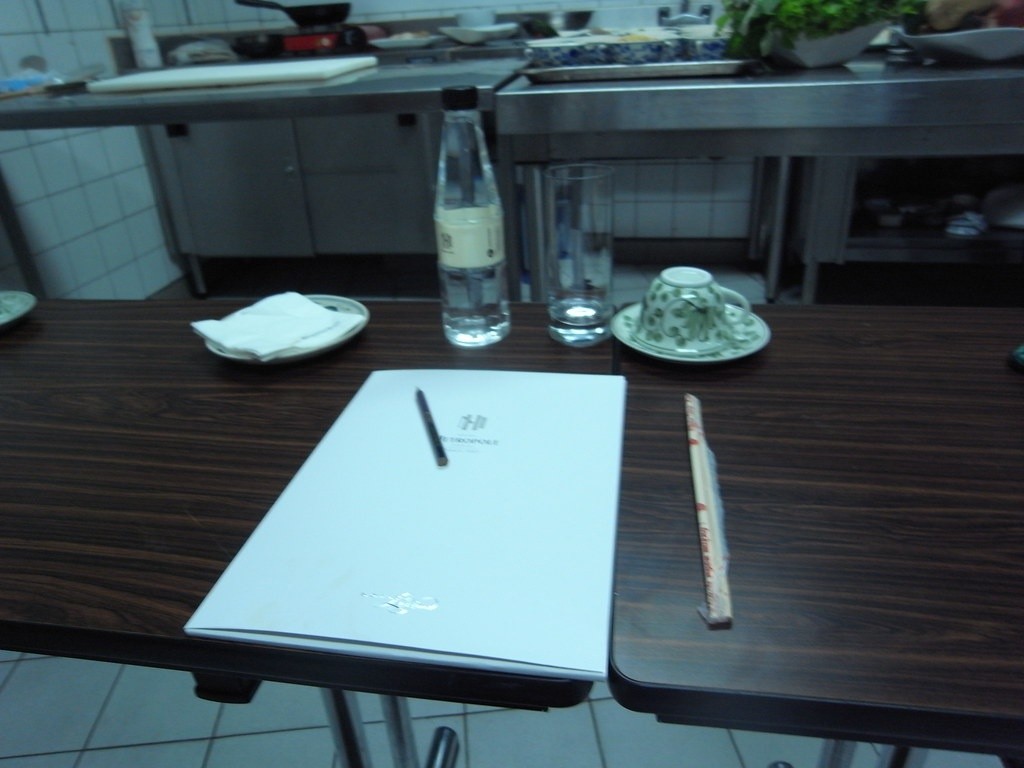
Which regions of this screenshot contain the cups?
[524,26,727,68]
[458,10,497,27]
[544,165,615,347]
[631,266,749,357]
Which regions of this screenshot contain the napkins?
[191,291,368,366]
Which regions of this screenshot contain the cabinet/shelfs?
[149,111,441,256]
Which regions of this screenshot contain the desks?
[0,289,616,768]
[611,299,1024,768]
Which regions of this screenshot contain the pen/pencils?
[413,386,448,467]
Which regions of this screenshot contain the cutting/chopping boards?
[88,55,377,81]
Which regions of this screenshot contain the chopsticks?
[686,394,733,624]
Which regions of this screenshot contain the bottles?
[433,86,513,347]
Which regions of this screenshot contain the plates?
[206,294,369,363]
[611,301,771,363]
[892,27,1024,60]
[368,35,446,49]
[0,291,38,325]
[440,22,519,43]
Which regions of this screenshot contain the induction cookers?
[270,27,366,54]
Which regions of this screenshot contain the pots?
[236,0,351,26]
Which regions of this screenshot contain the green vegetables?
[712,0,927,73]
